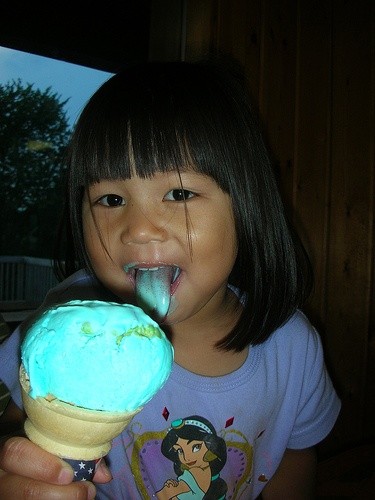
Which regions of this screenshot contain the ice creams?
[18,298,176,483]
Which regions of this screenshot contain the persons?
[1,60,343,499]
